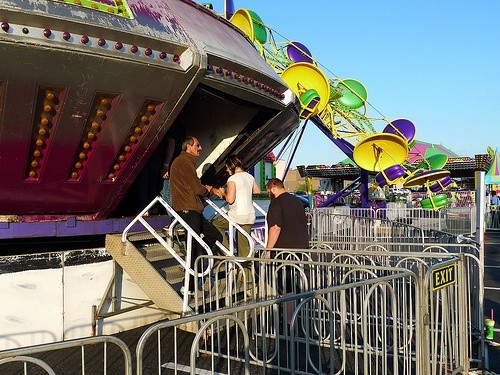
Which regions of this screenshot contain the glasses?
[193,144,199,148]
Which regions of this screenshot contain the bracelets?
[163,163,169,168]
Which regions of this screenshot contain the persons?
[170,136,223,296]
[212,155,261,282]
[136,126,185,217]
[262,178,309,325]
[465,191,500,228]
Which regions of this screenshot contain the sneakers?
[180,286,195,295]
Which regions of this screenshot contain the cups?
[484,319,495,339]
